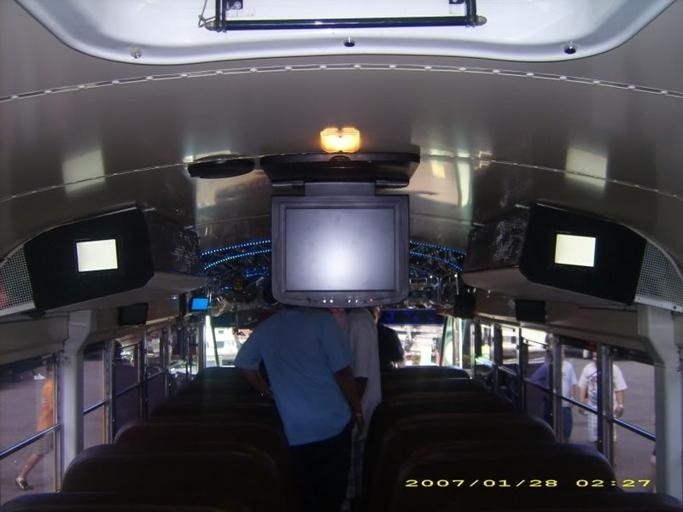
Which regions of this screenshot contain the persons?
[15,357,58,490]
[532,346,577,444]
[577,349,628,467]
[650,446,657,493]
[233,302,404,512]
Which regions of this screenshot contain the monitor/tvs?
[271,194,410,309]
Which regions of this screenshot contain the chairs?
[0,365,682,512]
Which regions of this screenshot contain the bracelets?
[618,404,623,407]
